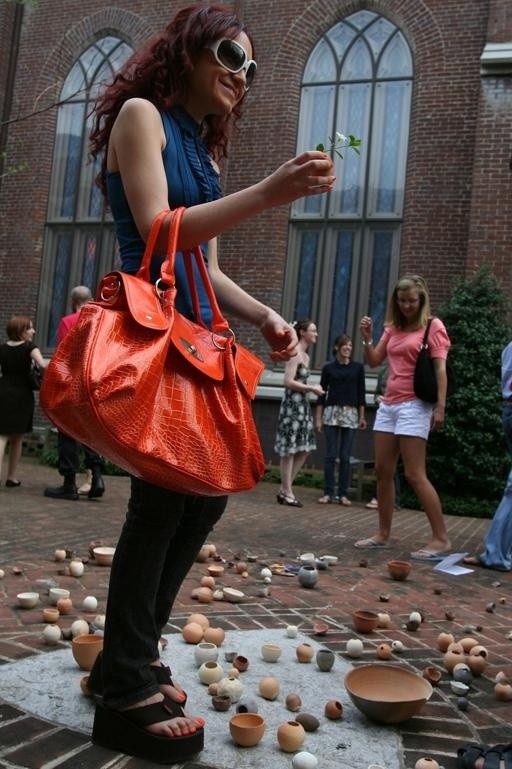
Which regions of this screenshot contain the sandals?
[276,490,379,509]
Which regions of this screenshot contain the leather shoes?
[6,479,21,487]
[43,475,106,500]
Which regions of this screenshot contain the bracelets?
[362,338,374,347]
[359,416,365,420]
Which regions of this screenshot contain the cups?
[0,539,119,694]
[158,541,511,766]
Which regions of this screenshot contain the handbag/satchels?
[413,316,459,405]
[38,205,269,501]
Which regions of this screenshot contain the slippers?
[463,554,511,573]
[410,547,452,560]
[80,649,188,709]
[354,537,391,548]
[90,692,206,763]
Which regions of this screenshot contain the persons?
[354,272,453,561]
[364,357,401,512]
[0,317,51,487]
[462,341,512,571]
[274,320,326,507]
[44,286,105,500]
[83,4,336,762]
[315,333,367,506]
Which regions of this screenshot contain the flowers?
[316,129,363,161]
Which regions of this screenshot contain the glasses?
[202,35,258,92]
